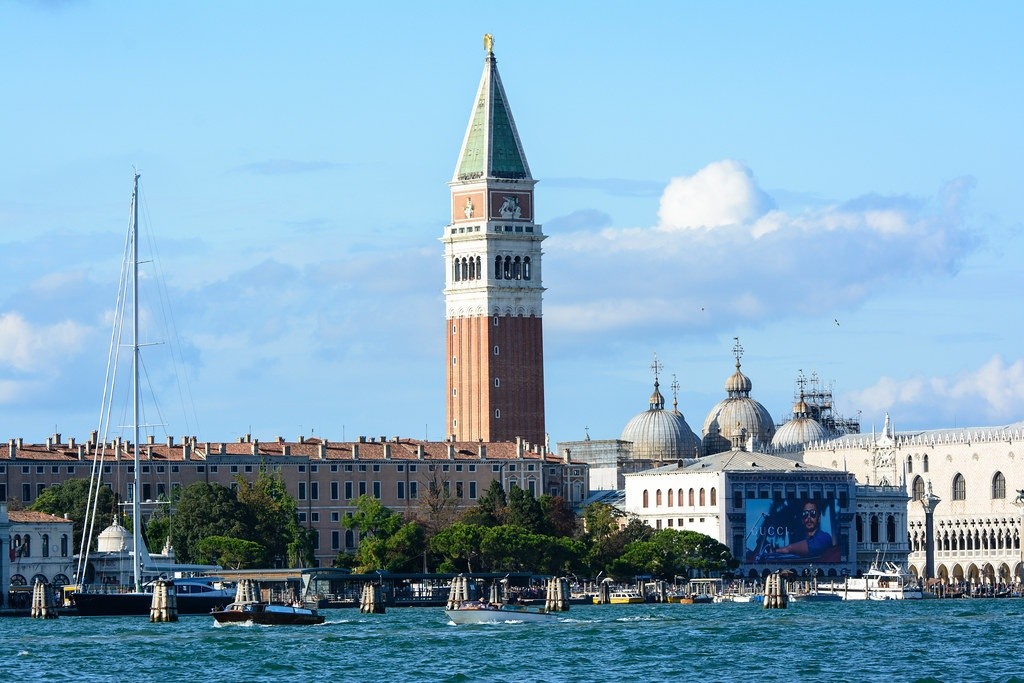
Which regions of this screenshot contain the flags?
[9,543,24,561]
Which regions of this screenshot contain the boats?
[942,587,1012,599]
[591,536,923,605]
[209,601,327,625]
[444,597,560,625]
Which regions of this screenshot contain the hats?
[479,597,485,602]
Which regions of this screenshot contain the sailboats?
[69,171,236,614]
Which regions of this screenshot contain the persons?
[767,502,834,559]
[879,574,1024,601]
[458,597,493,610]
[718,589,723,596]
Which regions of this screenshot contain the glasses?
[801,509,818,520]
